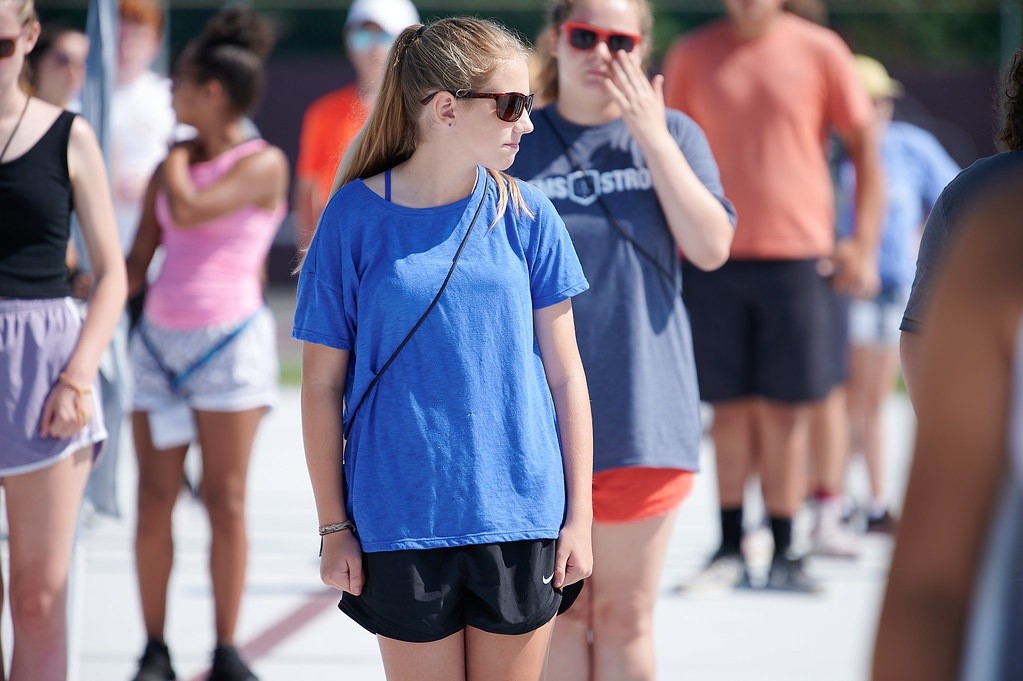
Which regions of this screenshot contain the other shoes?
[209,644,257,681]
[809,526,860,559]
[763,550,821,595]
[133,640,175,681]
[673,548,751,598]
[867,512,897,537]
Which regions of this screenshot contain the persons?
[293,0,1021,681]
[0,1,174,681]
[126,9,289,681]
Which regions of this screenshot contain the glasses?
[0,27,27,58]
[347,28,394,52]
[422,90,537,122]
[556,20,642,57]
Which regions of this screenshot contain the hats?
[851,53,905,99]
[341,0,420,42]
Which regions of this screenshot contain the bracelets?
[318,518,352,536]
[60,373,94,427]
